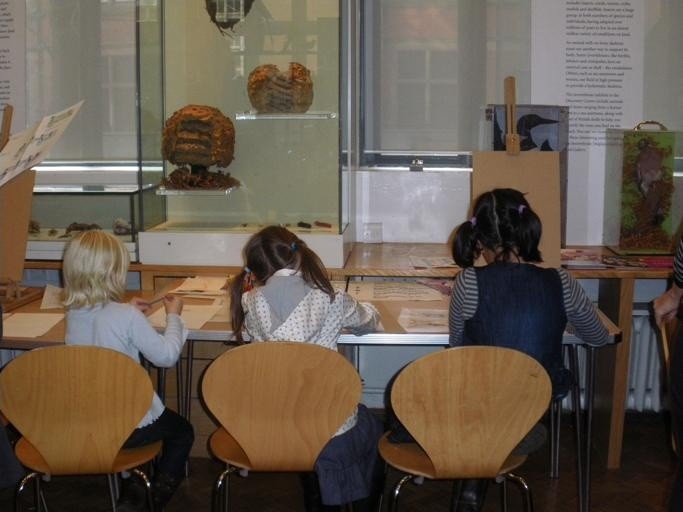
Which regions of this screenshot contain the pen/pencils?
[147,296,167,305]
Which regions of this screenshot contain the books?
[602,255,648,271]
[561,247,609,271]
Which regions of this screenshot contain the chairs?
[379,344,553,510]
[1,344,165,512]
[200,341,365,510]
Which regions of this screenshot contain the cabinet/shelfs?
[24,184,163,263]
[156,112,337,196]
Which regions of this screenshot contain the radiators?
[553,310,663,413]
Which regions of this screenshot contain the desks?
[344,241,674,469]
[1,285,169,402]
[131,278,624,510]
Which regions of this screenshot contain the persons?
[52,229,195,512]
[448,187,610,508]
[651,229,683,512]
[226,224,385,512]
